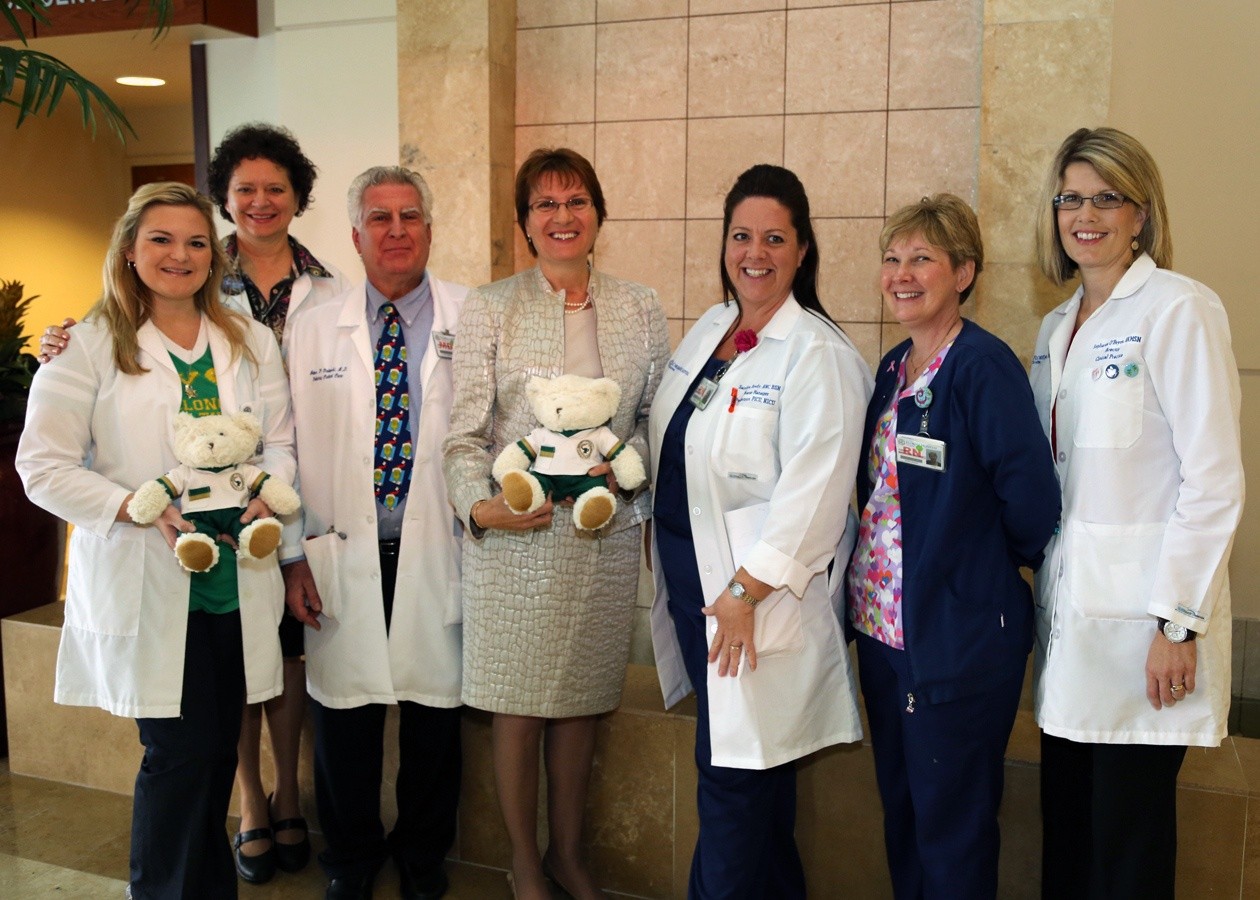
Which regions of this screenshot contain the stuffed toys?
[131,401,302,573]
[491,374,647,531]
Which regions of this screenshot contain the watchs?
[727,578,762,609]
[1156,615,1197,643]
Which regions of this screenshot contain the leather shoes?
[325,873,377,900]
[234,808,277,885]
[268,792,310,874]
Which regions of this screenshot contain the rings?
[1170,685,1184,692]
[730,645,741,650]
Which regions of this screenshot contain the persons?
[37,124,353,884]
[14,180,299,900]
[854,191,1063,900]
[1028,123,1249,900]
[639,163,877,899]
[259,164,482,899]
[440,144,673,899]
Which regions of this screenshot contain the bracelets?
[474,501,485,529]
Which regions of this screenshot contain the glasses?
[1054,192,1133,210]
[528,197,594,214]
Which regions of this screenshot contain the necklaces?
[561,294,591,314]
[910,322,958,374]
[149,311,200,399]
